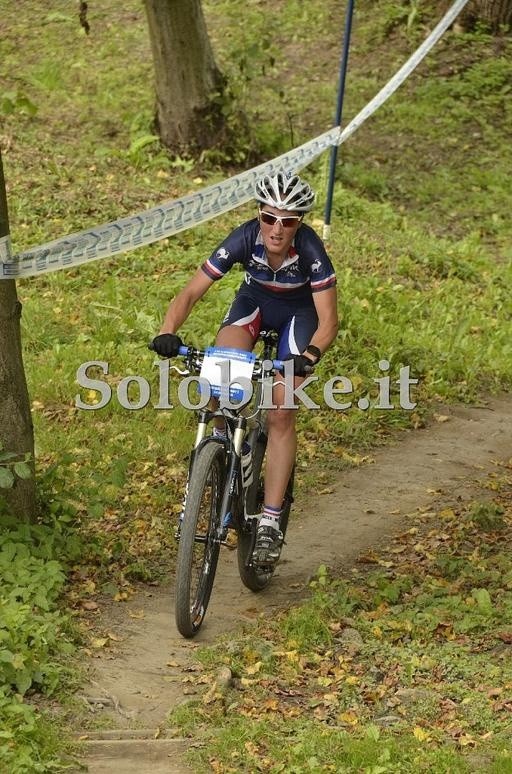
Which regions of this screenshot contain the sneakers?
[251,525,284,565]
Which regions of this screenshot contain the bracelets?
[303,345,321,365]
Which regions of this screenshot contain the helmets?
[254,171,316,212]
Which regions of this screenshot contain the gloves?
[153,333,187,358]
[279,353,313,378]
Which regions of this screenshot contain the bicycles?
[145,336,314,636]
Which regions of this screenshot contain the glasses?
[258,208,302,227]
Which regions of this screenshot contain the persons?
[154,175,339,566]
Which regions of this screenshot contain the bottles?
[240,439,254,490]
